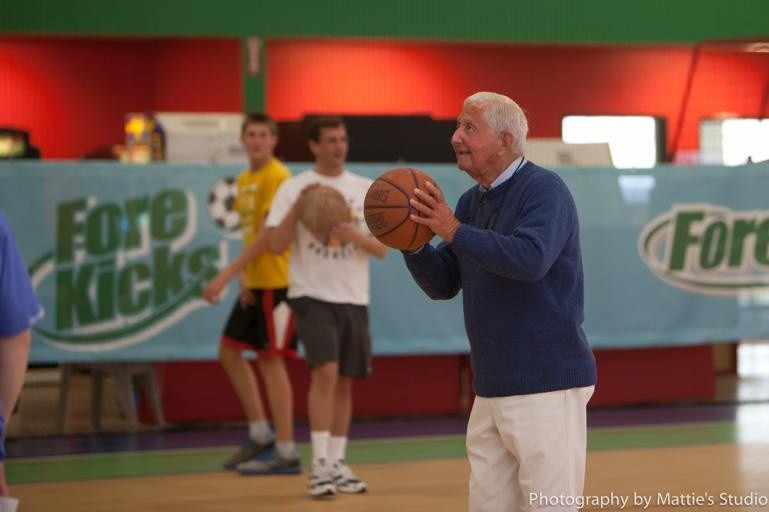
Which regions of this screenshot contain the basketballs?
[302,187,350,232]
[364,168,443,251]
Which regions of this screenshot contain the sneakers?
[236,447,304,475]
[223,431,275,469]
[307,464,336,496]
[330,458,368,493]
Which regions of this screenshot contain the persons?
[199,109,303,477]
[263,113,389,500]
[400,92,597,511]
[0,207,46,512]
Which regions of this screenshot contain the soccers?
[208,177,241,227]
